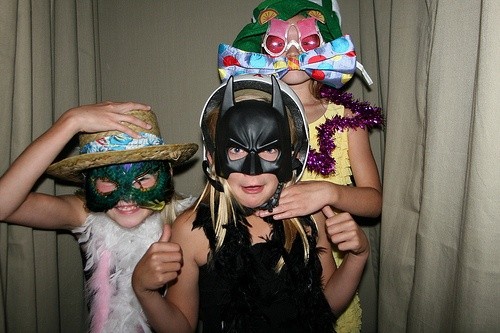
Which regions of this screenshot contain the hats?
[200,74,311,191]
[45,108,199,183]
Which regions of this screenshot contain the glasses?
[262,15,324,57]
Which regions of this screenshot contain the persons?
[131,76,368,332]
[1,101,197,332]
[215,0,385,332]
